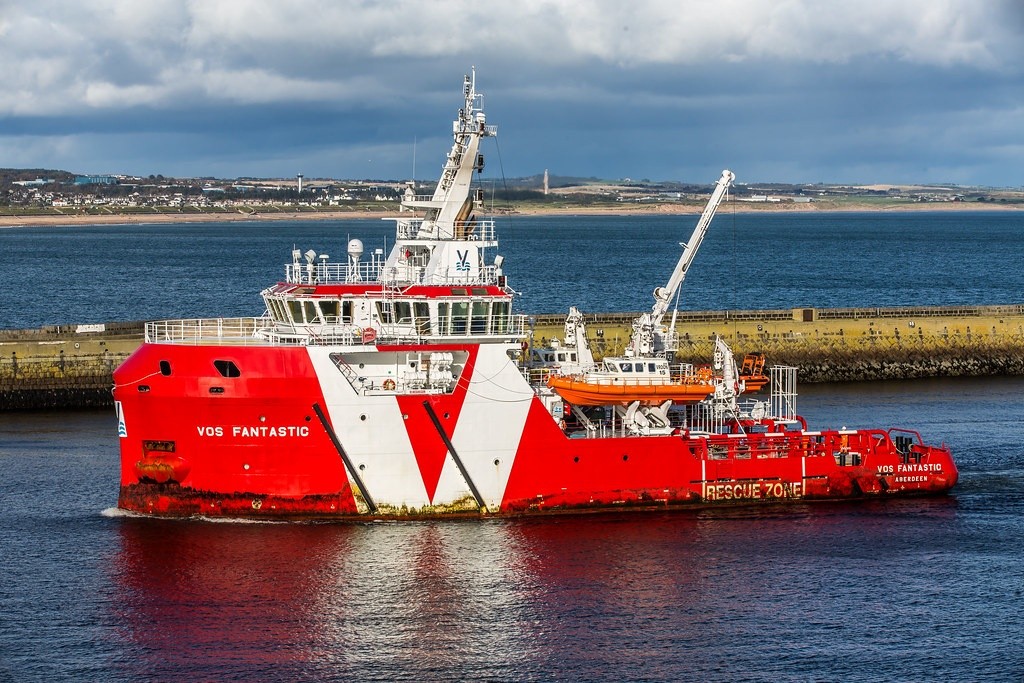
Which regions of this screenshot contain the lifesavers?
[363,328,375,340]
[383,379,395,391]
[544,374,555,385]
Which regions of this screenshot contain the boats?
[112,66,959,521]
[547,349,772,407]
